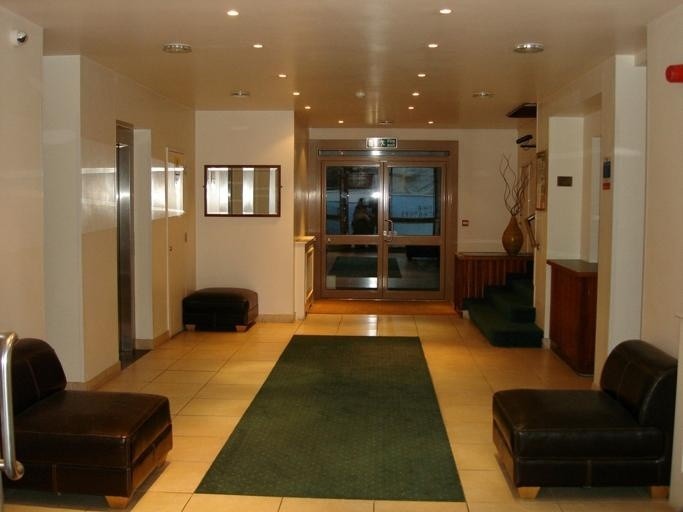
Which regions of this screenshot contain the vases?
[500,213,524,258]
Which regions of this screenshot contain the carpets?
[191,332,468,504]
[327,254,402,279]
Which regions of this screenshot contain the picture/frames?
[533,148,548,212]
[203,164,283,218]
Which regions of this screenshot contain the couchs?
[1,335,176,510]
[489,338,680,502]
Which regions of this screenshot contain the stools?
[180,286,260,334]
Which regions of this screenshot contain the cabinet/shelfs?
[454,251,532,318]
[546,257,596,378]
[294,235,315,318]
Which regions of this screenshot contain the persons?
[352,197,374,223]
